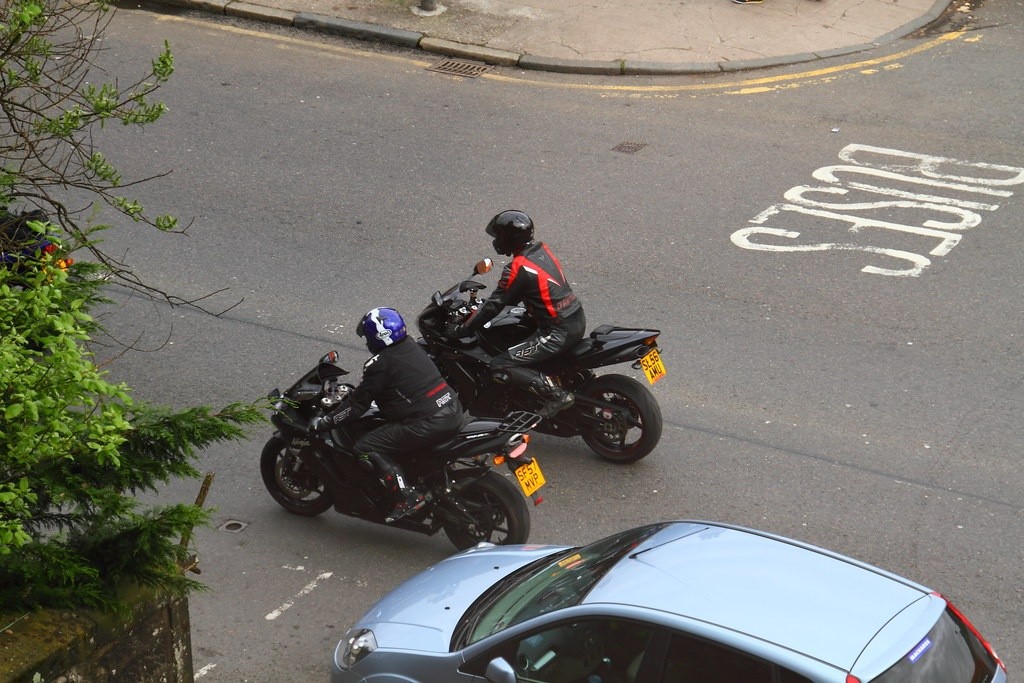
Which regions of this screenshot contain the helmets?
[356,307,406,356]
[485,210,534,257]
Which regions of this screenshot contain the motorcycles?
[260,349,548,552]
[416,258,668,465]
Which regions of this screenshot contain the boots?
[377,464,425,523]
[527,372,575,420]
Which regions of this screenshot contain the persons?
[307,307,463,522]
[443,210,585,420]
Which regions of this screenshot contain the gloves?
[481,299,486,303]
[308,415,336,433]
[442,323,463,346]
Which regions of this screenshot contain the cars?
[330,520,1010,683]
